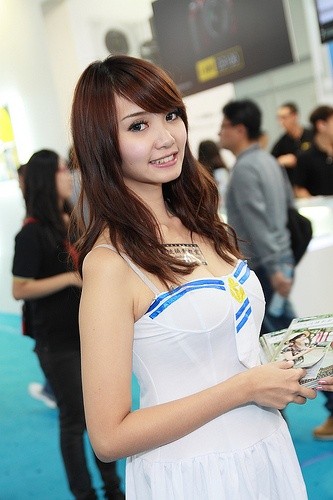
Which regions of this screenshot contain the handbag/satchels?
[286,205,313,268]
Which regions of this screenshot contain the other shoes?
[313,415,333,440]
[29,384,57,410]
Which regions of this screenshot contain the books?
[259,313,333,390]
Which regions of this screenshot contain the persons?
[197,101,333,208]
[11,149,126,500]
[218,99,297,337]
[17,147,96,408]
[71,55,333,500]
